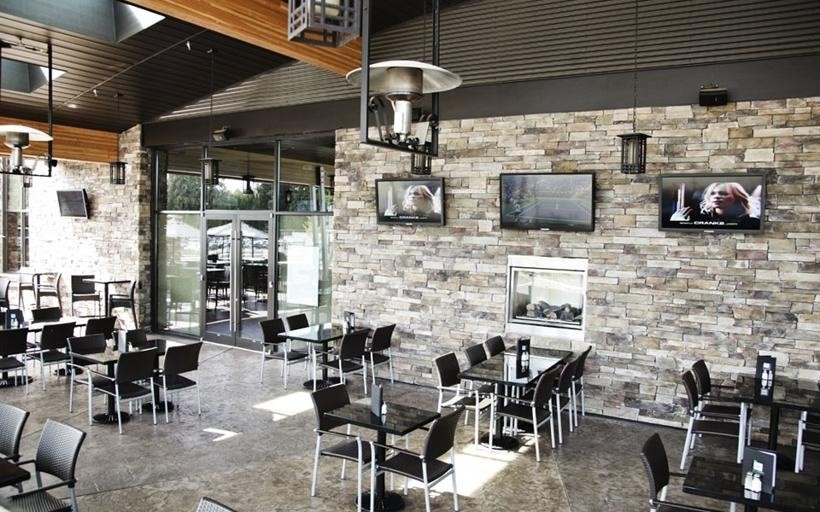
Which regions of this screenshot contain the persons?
[384,185,440,216]
[671,182,751,221]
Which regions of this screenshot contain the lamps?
[211,127,235,142]
[617,0,652,174]
[108,92,128,184]
[198,145,222,185]
[1,40,57,177]
[243,175,254,194]
[359,0,464,158]
[288,1,360,48]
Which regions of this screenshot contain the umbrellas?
[165,219,200,265]
[208,222,267,258]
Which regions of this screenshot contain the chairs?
[260,318,289,390]
[31,307,62,321]
[572,346,591,427]
[0,404,29,493]
[0,281,14,311]
[19,282,35,310]
[692,360,754,448]
[353,323,396,385]
[23,321,76,392]
[485,336,506,357]
[91,345,159,435]
[4,309,24,329]
[679,371,741,471]
[554,359,581,447]
[465,344,487,366]
[0,328,30,393]
[493,364,563,462]
[795,422,819,476]
[286,314,309,330]
[71,276,102,317]
[369,405,465,512]
[320,329,370,395]
[108,281,140,328]
[86,317,117,340]
[639,434,738,511]
[801,413,820,424]
[0,417,87,512]
[32,272,62,311]
[154,347,174,423]
[126,330,148,348]
[67,334,111,425]
[436,353,492,448]
[311,383,350,497]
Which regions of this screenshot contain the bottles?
[743,491,762,502]
[522,351,526,362]
[381,402,388,415]
[762,368,768,380]
[768,381,772,390]
[744,471,753,490]
[768,370,774,380]
[526,352,531,362]
[762,380,767,390]
[751,474,762,492]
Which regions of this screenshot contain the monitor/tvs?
[497,171,595,232]
[374,178,445,228]
[659,172,767,235]
[55,188,90,221]
